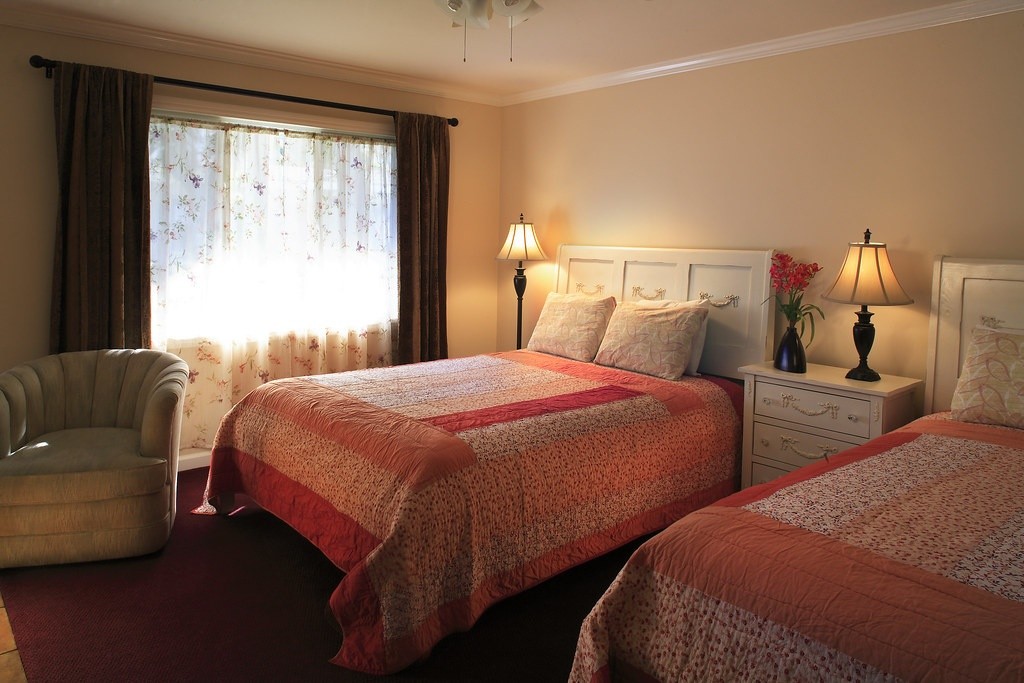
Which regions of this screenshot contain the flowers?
[761,251,824,350]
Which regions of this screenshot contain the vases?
[774,325,807,373]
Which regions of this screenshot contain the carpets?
[0,465,670,683]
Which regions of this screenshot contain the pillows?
[527,291,617,362]
[593,301,709,381]
[951,326,1024,430]
[638,298,709,377]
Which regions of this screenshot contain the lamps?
[435,0,544,63]
[820,227,915,382]
[494,213,549,350]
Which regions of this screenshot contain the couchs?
[0,349,189,568]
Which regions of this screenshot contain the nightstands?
[737,359,926,490]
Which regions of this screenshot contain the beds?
[568,254,1024,683]
[196,243,776,675]
